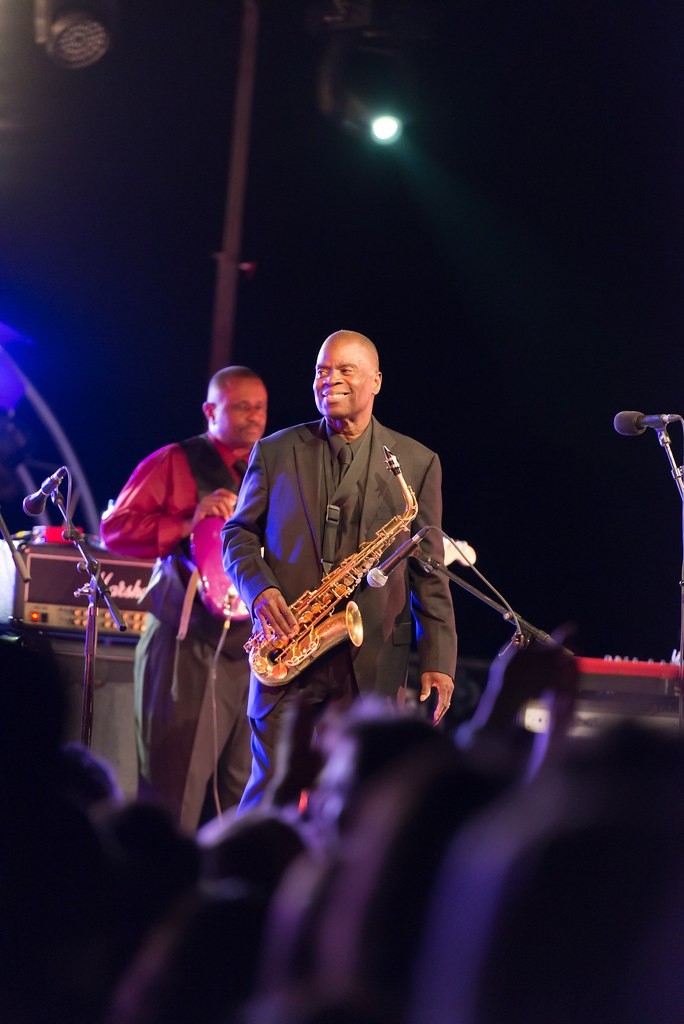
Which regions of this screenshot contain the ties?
[334,444,362,572]
[232,460,248,494]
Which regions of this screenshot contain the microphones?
[367,526,431,588]
[614,411,680,435]
[22,467,68,517]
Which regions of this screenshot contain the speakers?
[41,632,142,802]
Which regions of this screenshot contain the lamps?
[33,0,110,69]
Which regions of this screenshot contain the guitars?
[191,517,477,622]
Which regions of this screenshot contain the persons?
[100,366,269,833]
[220,330,458,814]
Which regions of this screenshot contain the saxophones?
[242,445,419,688]
